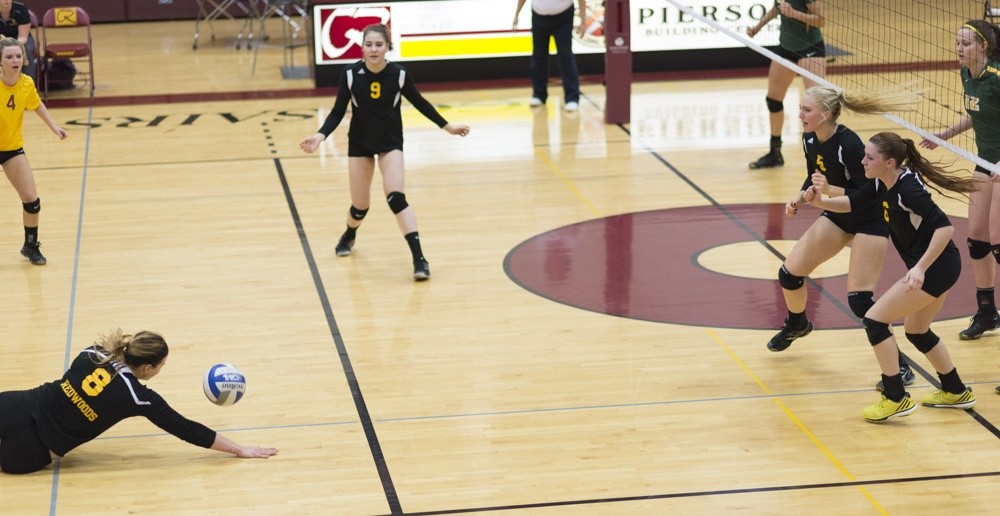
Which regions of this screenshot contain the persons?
[300,24,470,280]
[746,0,829,169]
[0,327,279,474]
[769,84,987,423]
[0,0,67,267]
[918,19,999,394]
[512,0,587,111]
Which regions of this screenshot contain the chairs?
[28,7,96,100]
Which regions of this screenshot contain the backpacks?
[35,55,76,91]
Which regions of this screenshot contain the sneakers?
[412,257,431,280]
[335,232,356,256]
[958,311,1000,340]
[20,242,46,265]
[864,392,916,423]
[749,151,784,169]
[876,364,916,391]
[767,317,813,352]
[921,386,976,409]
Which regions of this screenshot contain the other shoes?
[530,97,542,107]
[565,101,577,111]
[995,386,1000,393]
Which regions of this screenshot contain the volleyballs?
[202,362,246,407]
